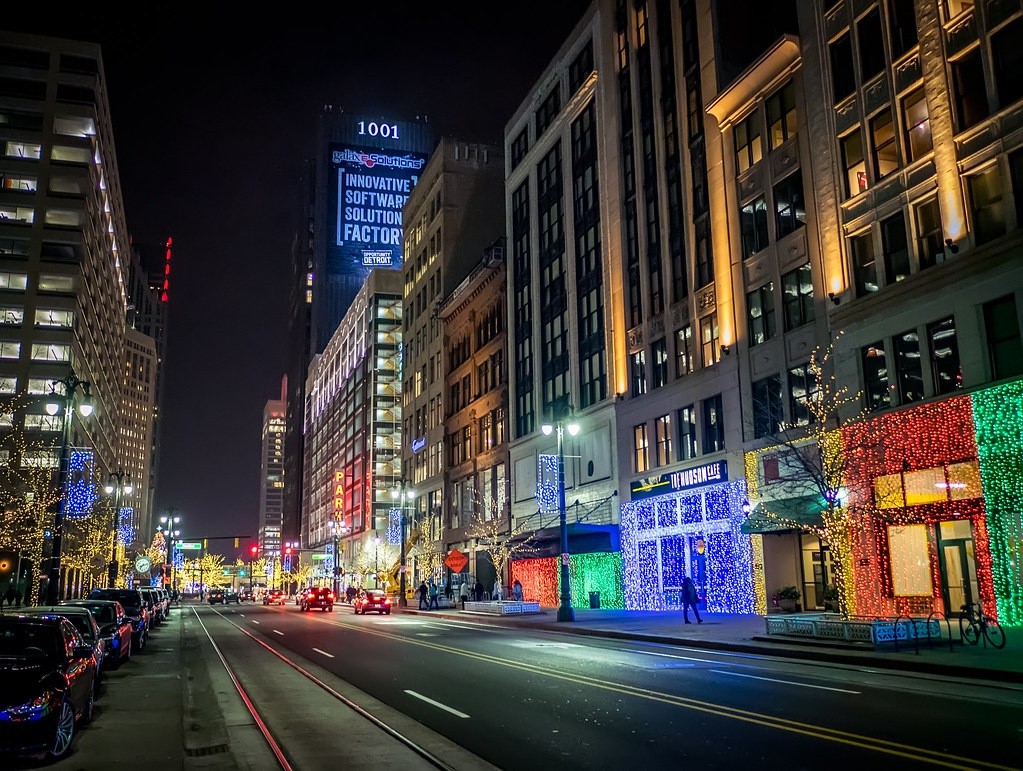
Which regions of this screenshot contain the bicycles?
[959,597,1007,649]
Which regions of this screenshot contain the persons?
[346,585,356,604]
[680,577,703,624]
[493,577,503,599]
[461,581,471,609]
[475,580,484,601]
[427,584,439,610]
[418,581,429,610]
[512,580,522,600]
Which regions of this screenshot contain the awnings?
[741,493,828,533]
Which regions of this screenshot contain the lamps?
[828,292,840,304]
[945,238,959,253]
[617,392,623,401]
[721,345,730,355]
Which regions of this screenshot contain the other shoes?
[685,621,691,624]
[698,620,703,624]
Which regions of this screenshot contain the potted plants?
[778,585,800,613]
[824,585,840,613]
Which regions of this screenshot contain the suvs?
[88,588,152,652]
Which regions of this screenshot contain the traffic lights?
[338,567,343,576]
[284,541,291,554]
[250,544,258,556]
[333,566,339,575]
[108,560,121,581]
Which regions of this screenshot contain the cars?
[0,611,99,762]
[59,600,134,672]
[206,589,256,605]
[18,606,107,700]
[354,589,391,617]
[295,588,308,606]
[139,586,182,630]
[300,586,334,612]
[262,589,286,605]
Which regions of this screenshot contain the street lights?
[42,375,96,604]
[391,474,416,606]
[539,397,581,627]
[160,504,180,596]
[327,512,347,603]
[366,529,383,588]
[104,466,132,587]
